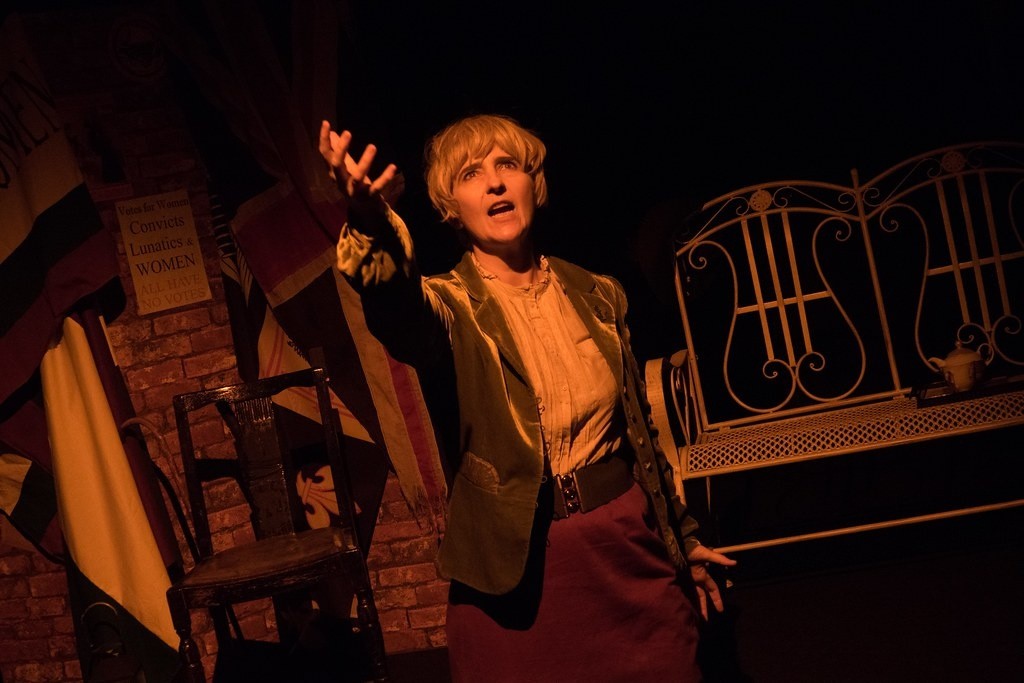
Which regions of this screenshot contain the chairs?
[167,365,392,683]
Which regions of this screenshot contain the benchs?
[643,138,1024,568]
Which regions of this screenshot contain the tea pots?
[928,341,995,392]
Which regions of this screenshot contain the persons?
[317,115,736,683]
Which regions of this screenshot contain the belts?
[534,444,638,520]
